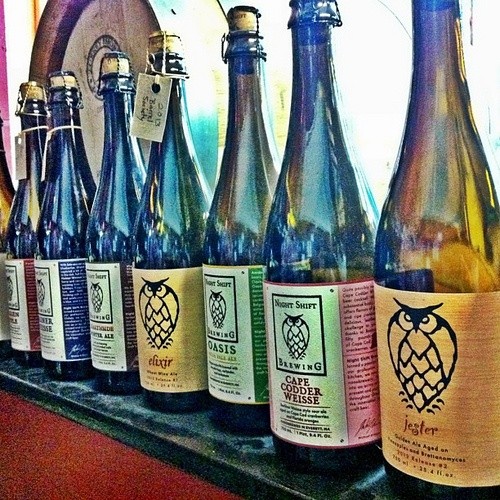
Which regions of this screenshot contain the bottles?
[372,0,499,500]
[4,80,50,371]
[33,69,101,382]
[87,51,154,399]
[201,6,282,436]
[0,102,16,359]
[129,33,212,418]
[263,0,381,476]
